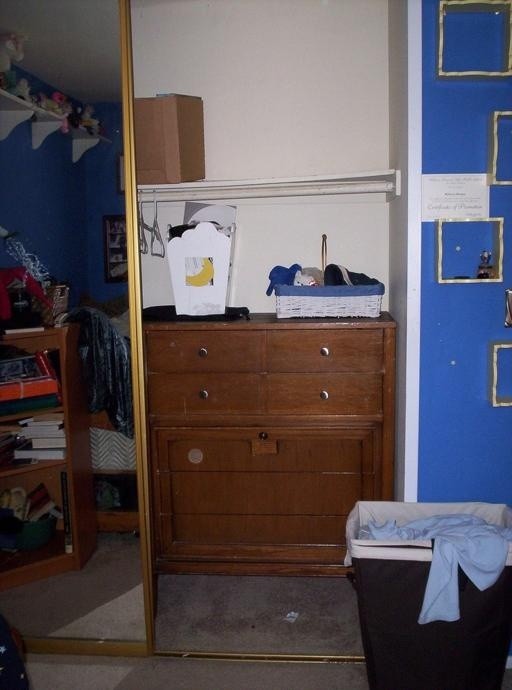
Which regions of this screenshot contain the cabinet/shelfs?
[433,0,512,407]
[0,327,99,589]
[140,323,395,576]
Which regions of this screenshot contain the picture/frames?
[102,216,128,284]
[116,152,125,194]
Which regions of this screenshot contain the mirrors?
[0,0,155,660]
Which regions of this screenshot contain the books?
[182,201,237,233]
[0,354,67,463]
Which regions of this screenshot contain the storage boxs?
[131,97,205,184]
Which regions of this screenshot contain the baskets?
[90,428,137,471]
[275,282,385,319]
[42,285,69,325]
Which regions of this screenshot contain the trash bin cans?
[345,501,511,690]
